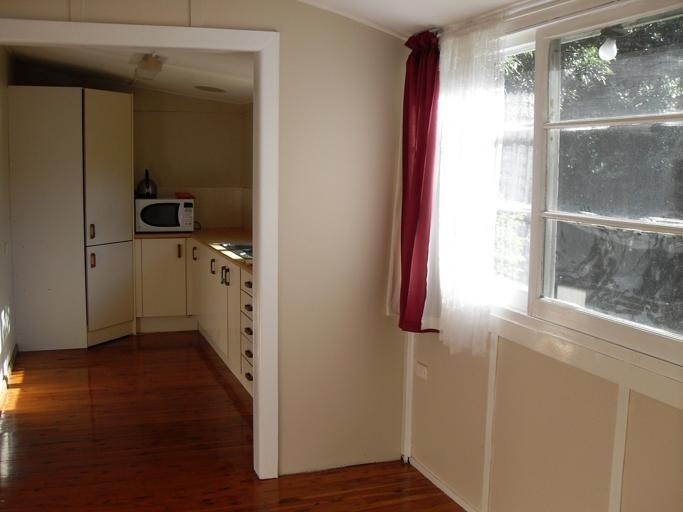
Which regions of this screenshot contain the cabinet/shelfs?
[215,258,240,378]
[140,240,188,317]
[238,269,252,397]
[190,240,216,344]
[9,85,135,351]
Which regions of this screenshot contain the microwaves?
[134,199,193,233]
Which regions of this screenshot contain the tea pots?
[138,167,156,198]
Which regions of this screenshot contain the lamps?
[134,57,162,82]
